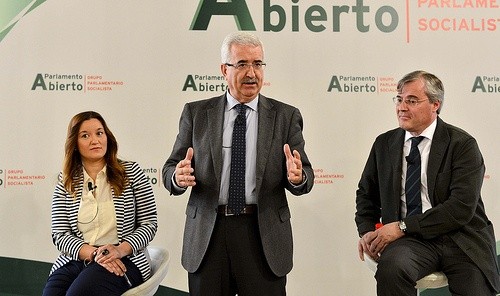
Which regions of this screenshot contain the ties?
[229,104,250,215]
[405,136,427,218]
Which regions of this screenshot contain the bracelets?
[90,248,99,262]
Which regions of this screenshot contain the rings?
[102,249,110,256]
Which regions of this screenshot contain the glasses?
[392,96,430,107]
[225,60,266,72]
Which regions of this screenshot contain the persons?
[161,29,315,296]
[353,69,500,296]
[40,110,158,296]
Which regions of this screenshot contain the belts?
[218,205,258,216]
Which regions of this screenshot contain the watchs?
[398,220,408,236]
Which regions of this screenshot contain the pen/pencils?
[123,273,131,286]
